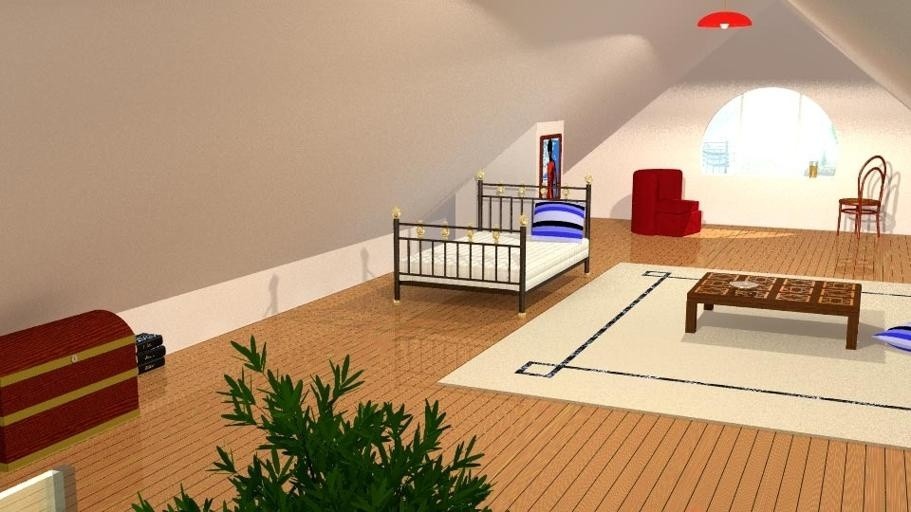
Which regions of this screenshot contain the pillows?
[872,324,911,353]
[532,201,585,244]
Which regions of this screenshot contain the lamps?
[697,0,751,31]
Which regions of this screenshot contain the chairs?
[836,155,888,240]
[631,168,703,238]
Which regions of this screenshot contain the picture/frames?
[539,132,563,200]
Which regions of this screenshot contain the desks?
[685,271,864,351]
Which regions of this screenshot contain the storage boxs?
[1,310,143,472]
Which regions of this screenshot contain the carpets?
[436,261,911,450]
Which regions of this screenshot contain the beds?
[393,169,594,318]
